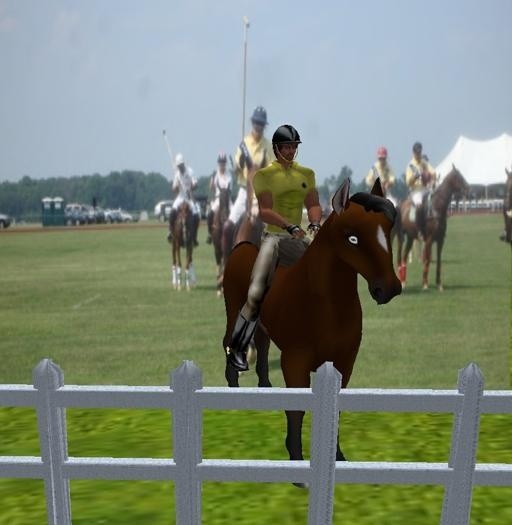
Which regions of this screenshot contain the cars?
[0,214,10,228]
[63,201,136,226]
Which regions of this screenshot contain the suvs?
[154,201,175,222]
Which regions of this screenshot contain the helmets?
[176,143,422,166]
[272,125,302,145]
[252,107,268,124]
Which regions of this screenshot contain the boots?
[225,313,259,370]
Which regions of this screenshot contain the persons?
[405,142,436,242]
[167,105,277,289]
[226,125,323,371]
[364,146,398,211]
